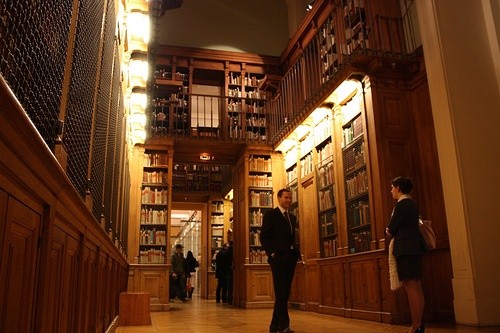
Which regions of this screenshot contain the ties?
[284,212,291,235]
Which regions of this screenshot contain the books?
[138,0,372,264]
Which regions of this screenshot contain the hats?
[176,244,184,248]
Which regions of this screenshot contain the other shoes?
[269,327,293,333]
[407,323,424,333]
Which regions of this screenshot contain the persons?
[384,177,424,333]
[260,189,301,333]
[169,240,234,305]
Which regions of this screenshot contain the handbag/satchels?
[418,219,438,251]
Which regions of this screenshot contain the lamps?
[125,0,153,149]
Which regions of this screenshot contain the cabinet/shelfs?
[128,0,435,325]
